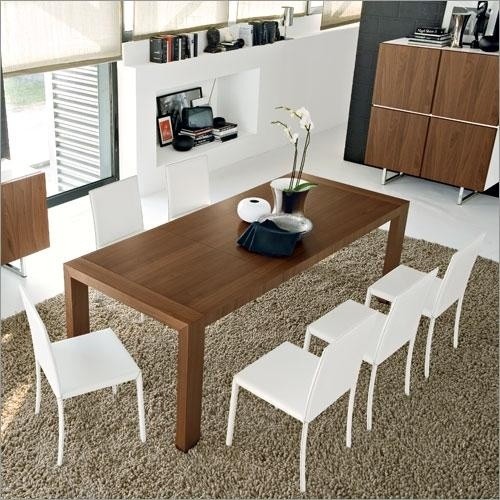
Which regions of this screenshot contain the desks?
[1,154,55,285]
[63,169,411,454]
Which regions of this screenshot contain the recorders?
[184,105,214,129]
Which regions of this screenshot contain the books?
[148,18,281,64]
[406,24,453,48]
[178,118,238,147]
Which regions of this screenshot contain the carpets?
[1,227,498,499]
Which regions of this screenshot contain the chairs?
[164,154,212,221]
[18,280,149,469]
[87,175,146,250]
[297,266,443,433]
[363,232,486,380]
[217,308,382,491]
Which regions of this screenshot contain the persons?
[173,93,189,118]
[161,95,182,132]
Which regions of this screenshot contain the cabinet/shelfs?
[363,33,499,204]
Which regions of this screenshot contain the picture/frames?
[155,87,204,134]
[156,113,176,149]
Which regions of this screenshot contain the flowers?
[271,104,320,189]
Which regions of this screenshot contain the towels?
[236,219,305,258]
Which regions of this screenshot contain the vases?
[447,8,473,49]
[271,176,315,242]
